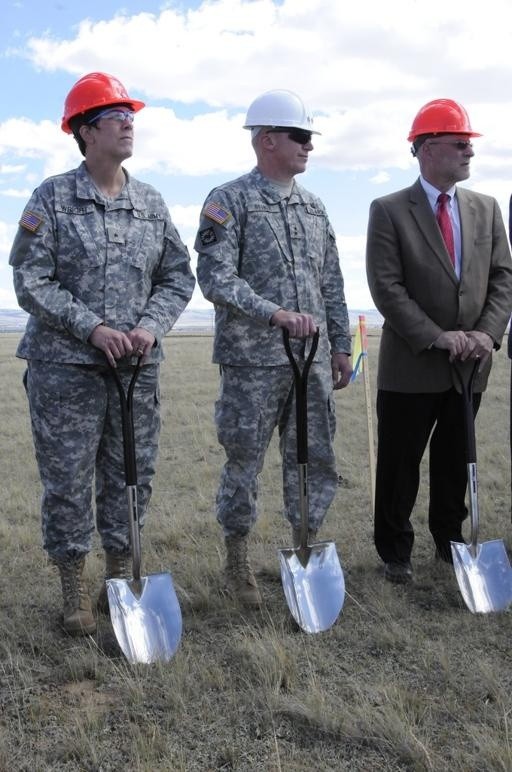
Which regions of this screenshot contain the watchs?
[364,100,512,586]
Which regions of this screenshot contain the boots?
[225,537,262,609]
[99,551,131,614]
[293,528,316,547]
[58,558,96,637]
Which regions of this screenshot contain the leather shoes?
[436,540,467,562]
[386,562,414,584]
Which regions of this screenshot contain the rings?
[475,354,482,358]
[136,348,145,356]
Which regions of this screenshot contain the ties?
[437,194,455,272]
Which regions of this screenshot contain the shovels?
[450,353,512,614]
[277,326,345,633]
[107,354,183,666]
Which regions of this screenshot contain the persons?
[7,70,194,636]
[193,91,354,612]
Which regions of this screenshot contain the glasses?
[266,129,311,144]
[429,142,473,149]
[85,108,134,122]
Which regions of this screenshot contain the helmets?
[243,89,322,135]
[407,99,482,140]
[61,72,145,134]
